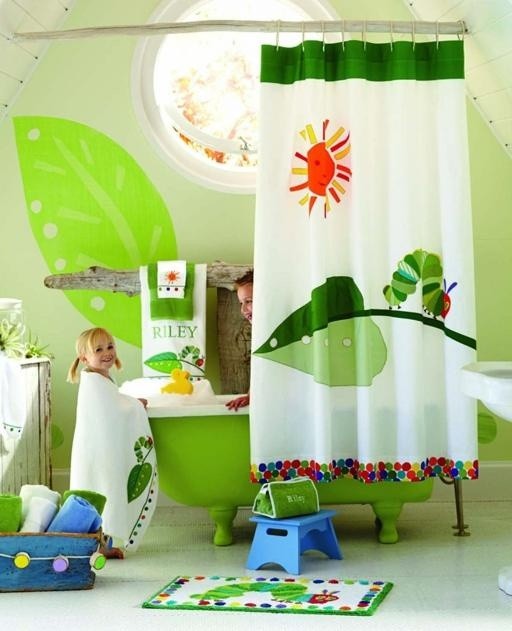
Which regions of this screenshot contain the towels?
[1,353,28,453]
[146,261,195,321]
[138,261,209,382]
[156,260,187,299]
[67,366,161,553]
[1,483,108,535]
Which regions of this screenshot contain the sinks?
[464,360,512,427]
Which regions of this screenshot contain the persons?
[66,328,148,559]
[224,271,253,412]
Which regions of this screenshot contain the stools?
[246,507,345,576]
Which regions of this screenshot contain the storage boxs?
[0,532,101,594]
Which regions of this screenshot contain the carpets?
[141,575,395,618]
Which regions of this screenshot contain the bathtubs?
[144,393,433,546]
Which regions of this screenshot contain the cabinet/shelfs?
[0,350,54,496]
